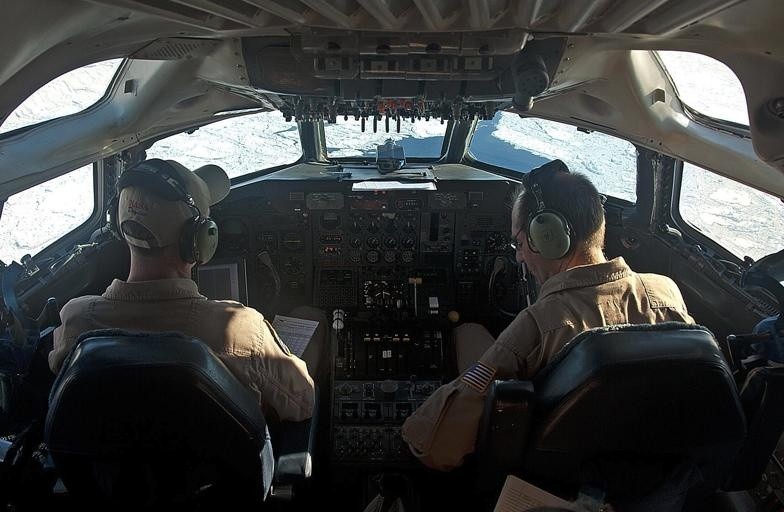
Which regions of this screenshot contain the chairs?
[465,320,752,511]
[42,327,316,511]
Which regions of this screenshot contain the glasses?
[509,223,526,251]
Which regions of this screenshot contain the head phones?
[105,158,220,265]
[521,159,576,260]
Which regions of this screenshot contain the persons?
[47,157,332,421]
[400,159,699,471]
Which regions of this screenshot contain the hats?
[119,158,230,249]
